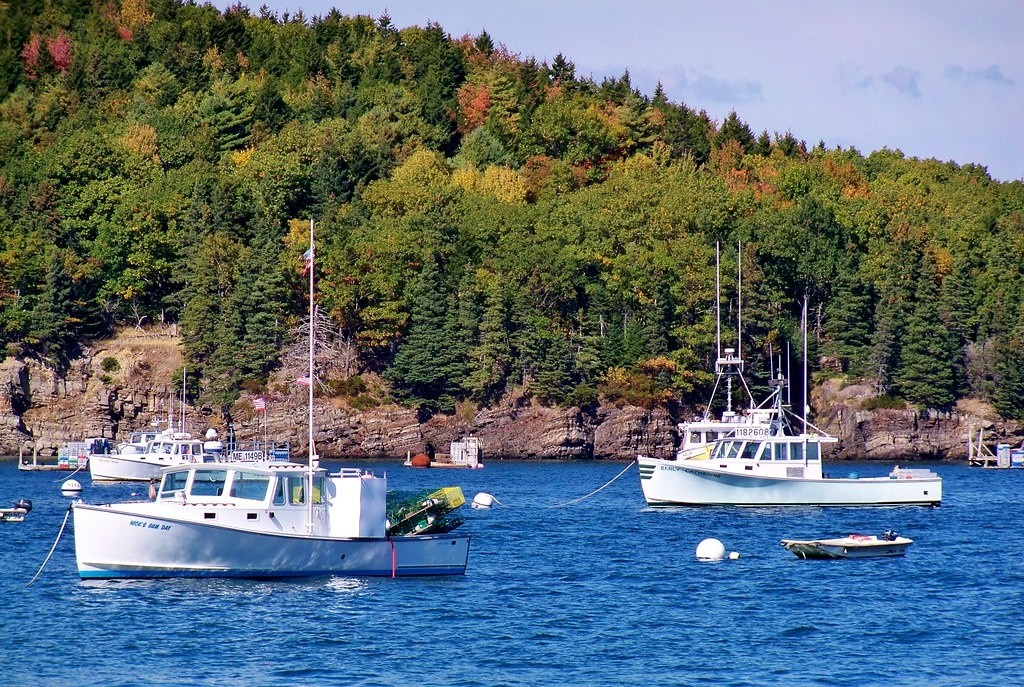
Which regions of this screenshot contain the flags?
[303,243,315,275]
[297,373,315,386]
[801,306,805,330]
[253,398,266,410]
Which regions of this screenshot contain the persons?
[733,446,749,458]
[184,446,194,459]
[221,417,236,455]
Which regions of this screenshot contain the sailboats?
[72,220,471,587]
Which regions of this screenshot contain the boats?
[637,239,943,506]
[780,529,914,560]
[88,366,290,482]
